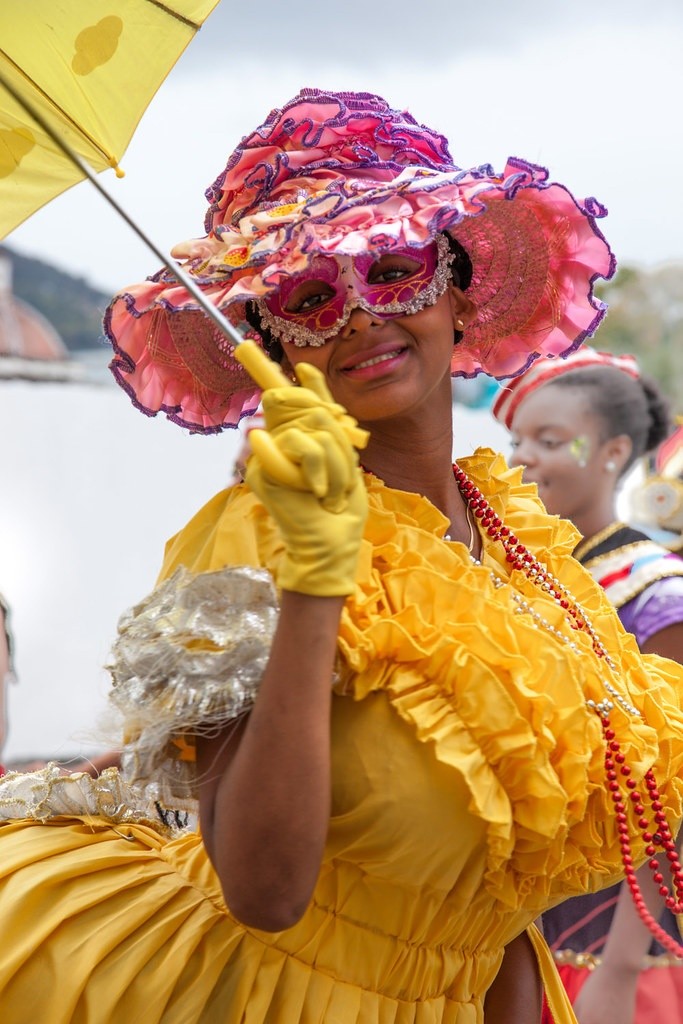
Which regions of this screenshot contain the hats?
[488,343,640,431]
[103,87,617,440]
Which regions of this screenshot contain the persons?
[489,348,682,1024]
[1,86,683,1024]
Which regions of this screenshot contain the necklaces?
[353,447,682,961]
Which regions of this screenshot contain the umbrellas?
[1,1,295,393]
